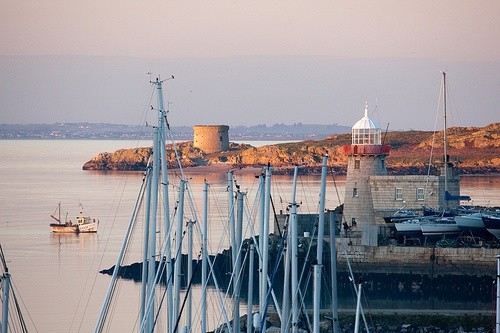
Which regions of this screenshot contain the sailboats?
[76,203,98,233]
[49,202,79,235]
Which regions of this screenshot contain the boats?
[382,69,500,244]
